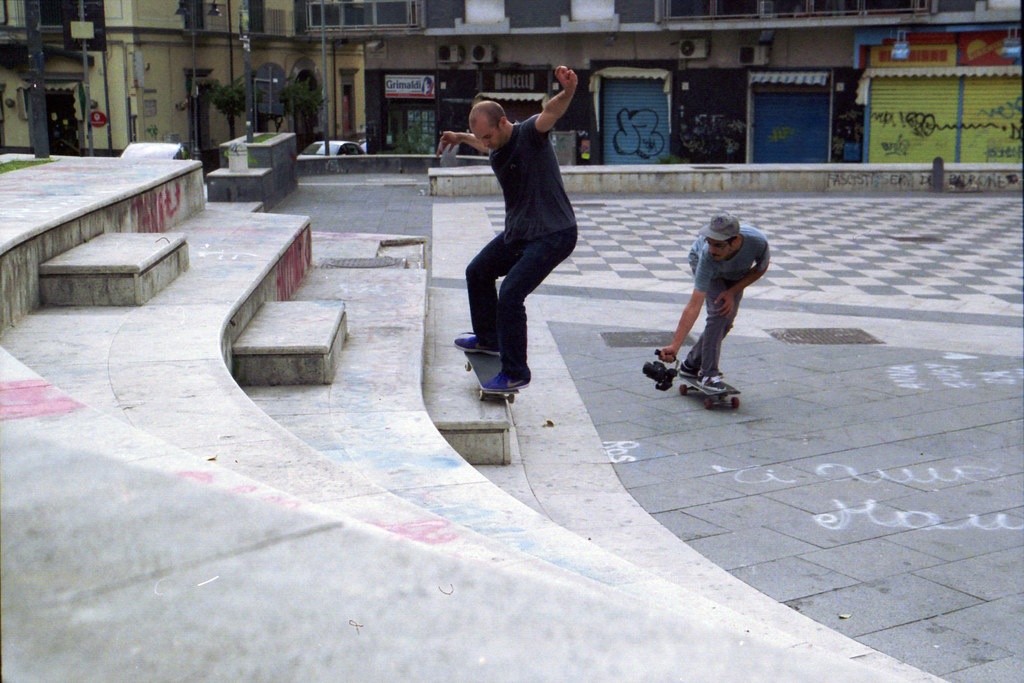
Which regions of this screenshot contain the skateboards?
[678,368,741,410]
[459,331,530,405]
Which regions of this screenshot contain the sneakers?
[479,371,531,391]
[678,359,724,378]
[696,369,726,392]
[453,335,501,356]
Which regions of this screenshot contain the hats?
[698,213,740,241]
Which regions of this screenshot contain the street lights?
[254,77,278,133]
[191,0,223,160]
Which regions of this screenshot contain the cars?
[297,140,365,159]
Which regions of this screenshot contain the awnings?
[854,65,1022,105]
[471,91,549,109]
[747,70,828,87]
[589,66,672,93]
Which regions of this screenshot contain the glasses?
[703,237,728,248]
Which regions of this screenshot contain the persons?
[436,65,578,391]
[658,213,770,391]
